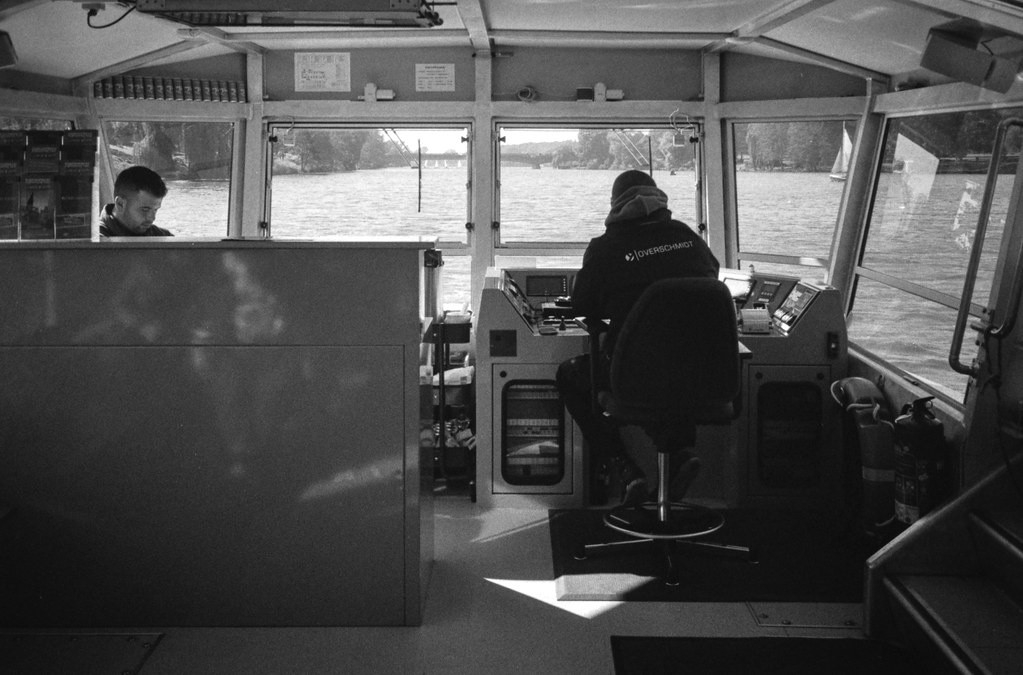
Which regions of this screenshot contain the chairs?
[573,276,765,583]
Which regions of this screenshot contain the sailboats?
[828,120,853,182]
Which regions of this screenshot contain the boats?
[670,169,676,175]
[410,160,419,169]
[528,153,544,169]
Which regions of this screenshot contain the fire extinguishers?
[890,396,945,539]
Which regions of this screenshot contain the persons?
[554,168,720,507]
[98,166,174,236]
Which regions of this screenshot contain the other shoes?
[668,452,701,503]
[614,461,646,510]
